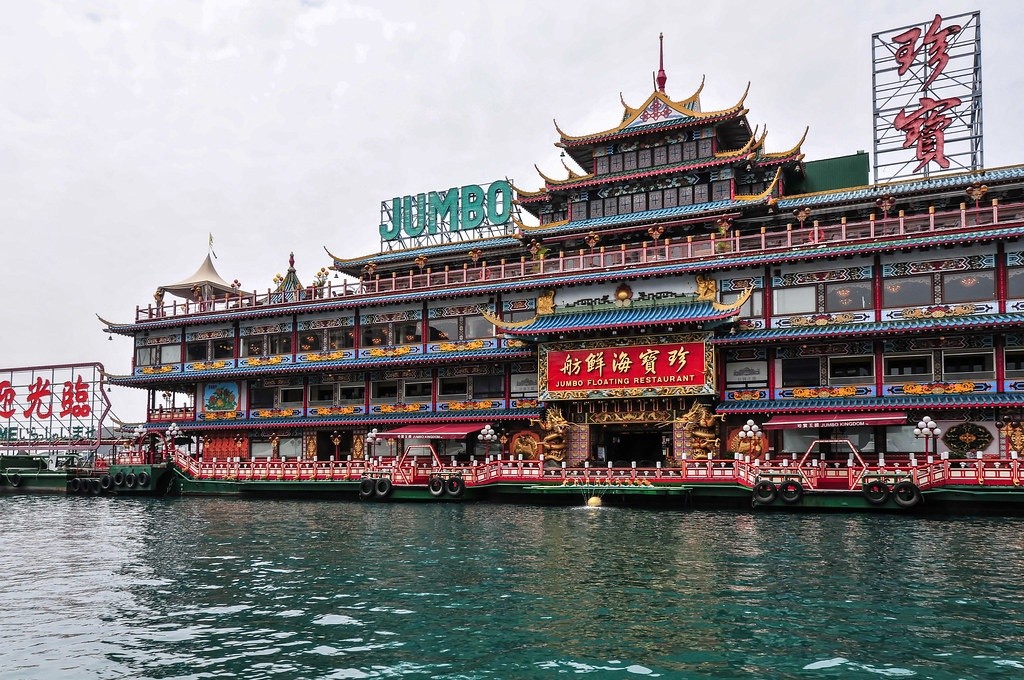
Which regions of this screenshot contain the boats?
[0,11,1023,518]
[520,479,693,512]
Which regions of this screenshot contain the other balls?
[587,496,602,507]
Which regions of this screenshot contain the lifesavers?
[71,478,81,492]
[894,479,919,508]
[754,480,778,505]
[79,479,92,493]
[90,479,102,492]
[429,477,445,496]
[779,479,804,504]
[101,473,113,491]
[358,476,376,498]
[10,472,21,488]
[137,472,151,486]
[122,471,137,488]
[445,475,464,497]
[862,478,891,506]
[374,477,392,499]
[113,471,125,486]
